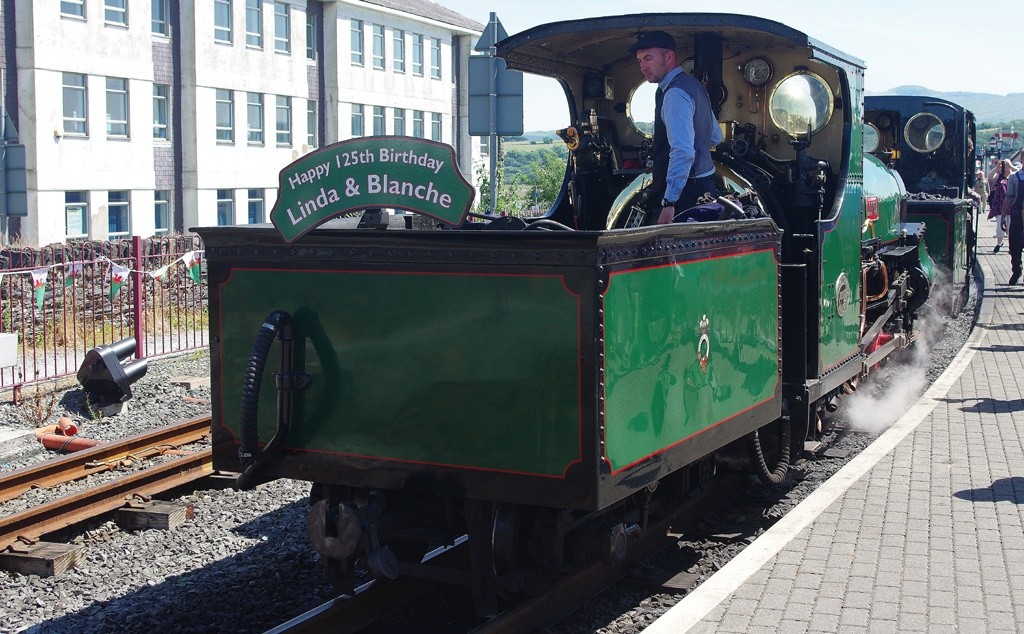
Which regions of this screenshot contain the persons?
[1000,147,1024,286]
[975,172,990,215]
[632,31,723,226]
[987,159,1023,253]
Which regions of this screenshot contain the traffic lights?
[76,336,148,405]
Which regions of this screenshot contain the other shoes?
[1009,274,1019,285]
[994,243,1003,253]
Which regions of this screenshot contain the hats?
[629,30,676,51]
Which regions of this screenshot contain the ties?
[655,88,663,106]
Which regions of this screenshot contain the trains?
[187,8,984,623]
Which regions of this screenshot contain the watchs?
[660,198,677,207]
